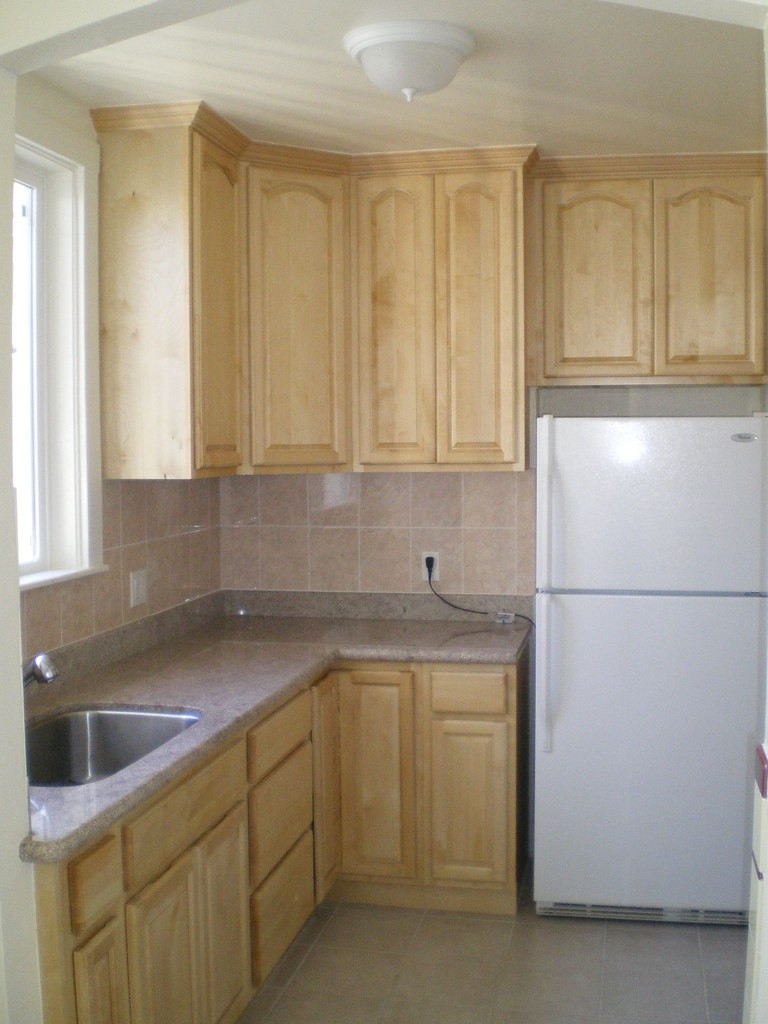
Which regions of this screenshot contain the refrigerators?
[533,413,768,923]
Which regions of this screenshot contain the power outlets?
[421,552,439,581]
[130,570,147,608]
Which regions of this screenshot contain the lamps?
[344,20,478,102]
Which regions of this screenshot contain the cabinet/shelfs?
[33,647,529,1024]
[90,102,768,481]
